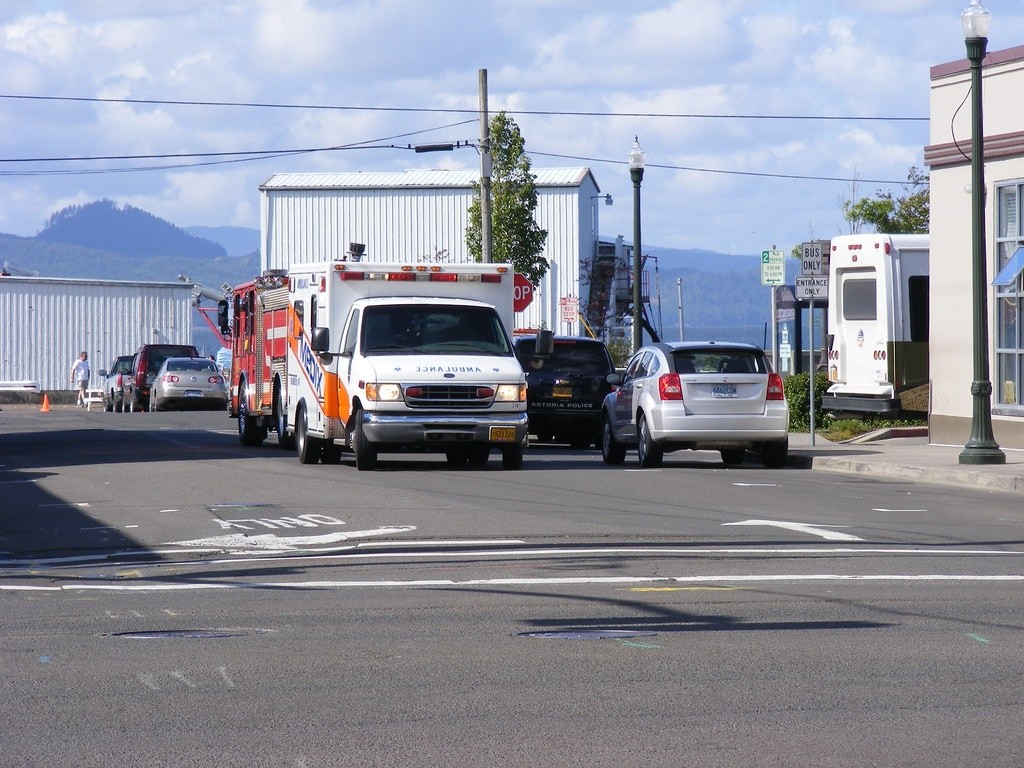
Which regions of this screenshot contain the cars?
[147,357,228,412]
[515,334,616,451]
[599,341,791,469]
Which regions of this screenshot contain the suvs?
[99,355,136,413]
[120,343,203,412]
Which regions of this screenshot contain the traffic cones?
[40,393,52,411]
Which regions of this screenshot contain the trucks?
[821,231,929,418]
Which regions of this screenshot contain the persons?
[70,352,90,408]
[208,355,218,372]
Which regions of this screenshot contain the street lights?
[959,0,1011,465]
[627,132,646,357]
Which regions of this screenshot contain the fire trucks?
[215,267,295,448]
[285,261,556,470]
